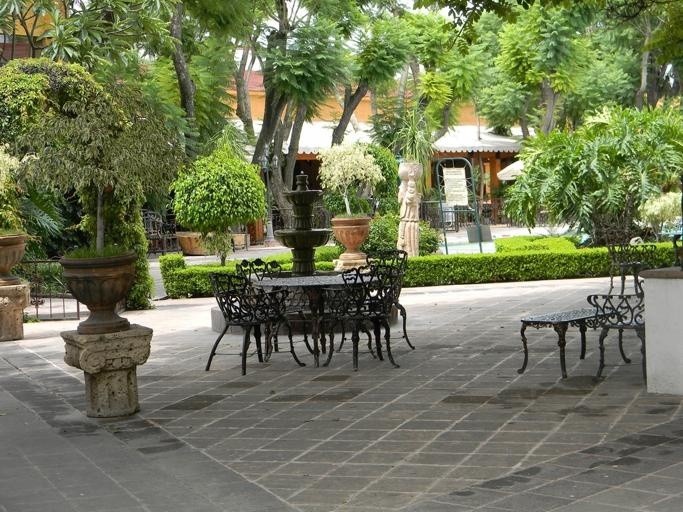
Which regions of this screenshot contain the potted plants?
[0,233,26,285]
[60,247,137,334]
[331,213,371,260]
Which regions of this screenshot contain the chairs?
[206,252,415,375]
[140,208,180,259]
[587,235,683,379]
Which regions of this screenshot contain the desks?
[518,308,620,378]
